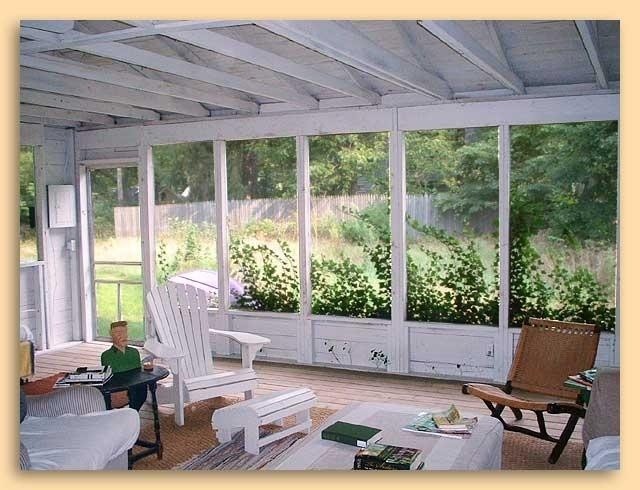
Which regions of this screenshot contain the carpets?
[120,393,587,468]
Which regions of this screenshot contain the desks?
[81,366,169,470]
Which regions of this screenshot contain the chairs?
[19,382,141,472]
[142,280,272,428]
[462,316,604,463]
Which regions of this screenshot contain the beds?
[278,401,504,469]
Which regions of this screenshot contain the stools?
[211,385,316,455]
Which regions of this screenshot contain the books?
[564,366,597,390]
[53,362,113,387]
[353,443,424,470]
[322,420,383,448]
[433,403,467,429]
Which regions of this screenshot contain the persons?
[102,321,140,372]
